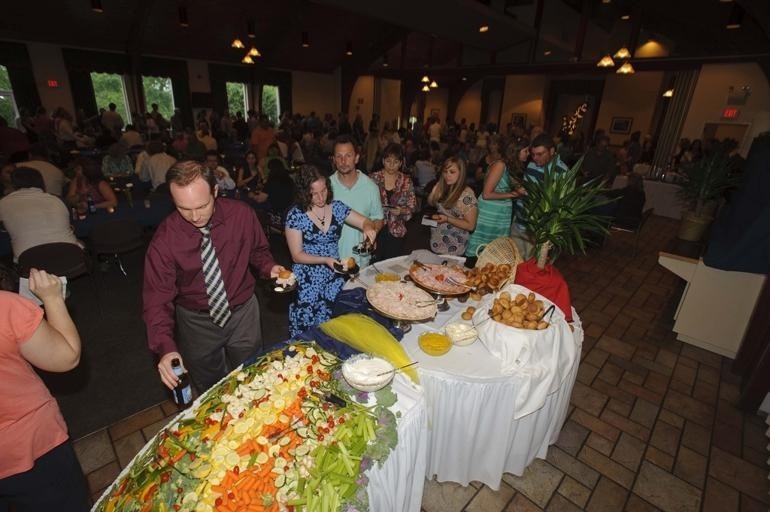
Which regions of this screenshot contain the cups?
[360,254,369,277]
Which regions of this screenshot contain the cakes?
[276,270,297,289]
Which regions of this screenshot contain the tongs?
[538,305,556,328]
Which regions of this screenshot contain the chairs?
[602,208,655,258]
[18,242,103,315]
[415,179,438,225]
[129,144,144,164]
[90,219,145,278]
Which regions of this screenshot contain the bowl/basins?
[418,331,452,356]
[343,356,395,391]
[446,321,478,346]
[375,271,401,283]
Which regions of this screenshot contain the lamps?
[420,74,439,92]
[302,32,309,48]
[91,0,104,13]
[596,44,636,74]
[230,17,261,65]
[177,5,189,28]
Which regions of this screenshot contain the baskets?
[476,237,524,288]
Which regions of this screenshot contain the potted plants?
[669,138,745,242]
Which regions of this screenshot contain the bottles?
[170,357,193,408]
[70,193,78,223]
[87,192,97,214]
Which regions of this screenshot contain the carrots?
[211,402,302,512]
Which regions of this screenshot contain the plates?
[271,278,298,294]
[333,260,360,275]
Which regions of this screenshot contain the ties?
[200,226,232,328]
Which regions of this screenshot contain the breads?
[462,312,471,320]
[467,307,475,315]
[340,256,356,271]
[493,291,549,330]
[452,263,511,301]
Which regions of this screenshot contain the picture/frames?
[609,117,633,135]
[511,112,527,128]
[431,109,439,116]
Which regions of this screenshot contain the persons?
[143,159,296,395]
[1,268,92,511]
[1,102,575,372]
[579,128,708,196]
